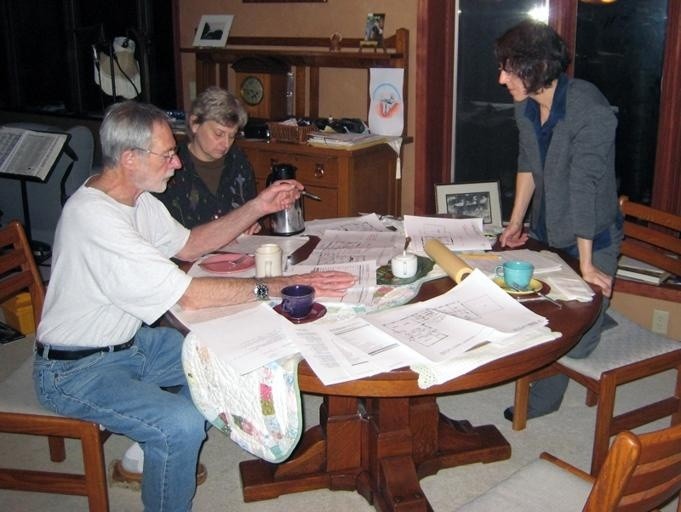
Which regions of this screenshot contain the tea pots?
[391,252,418,279]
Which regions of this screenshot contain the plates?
[273,305,327,325]
[493,277,544,295]
[200,254,256,275]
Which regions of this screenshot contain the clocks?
[231,56,284,120]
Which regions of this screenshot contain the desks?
[168,215,604,512]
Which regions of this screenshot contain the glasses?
[130,144,180,163]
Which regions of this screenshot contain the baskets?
[265,120,319,144]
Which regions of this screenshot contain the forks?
[508,281,563,308]
[198,255,246,266]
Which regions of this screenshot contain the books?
[308,132,388,152]
[617,255,670,285]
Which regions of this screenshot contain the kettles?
[258,163,305,236]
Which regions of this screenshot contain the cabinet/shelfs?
[180,27,413,217]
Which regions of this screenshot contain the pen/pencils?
[466,251,503,262]
[280,180,323,203]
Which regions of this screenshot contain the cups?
[495,261,534,290]
[280,284,315,321]
[255,244,281,278]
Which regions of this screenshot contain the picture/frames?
[192,13,235,49]
[364,13,386,44]
[434,180,504,235]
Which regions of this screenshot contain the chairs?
[454,422,681,512]
[512,194,680,483]
[0,221,114,512]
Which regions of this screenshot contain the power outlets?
[649,308,669,335]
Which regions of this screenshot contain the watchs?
[253,275,270,302]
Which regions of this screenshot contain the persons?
[493,19,622,422]
[150,88,263,247]
[34,101,359,512]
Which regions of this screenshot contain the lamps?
[93,26,151,103]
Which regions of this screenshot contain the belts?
[34,337,135,361]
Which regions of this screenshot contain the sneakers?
[112,457,207,492]
